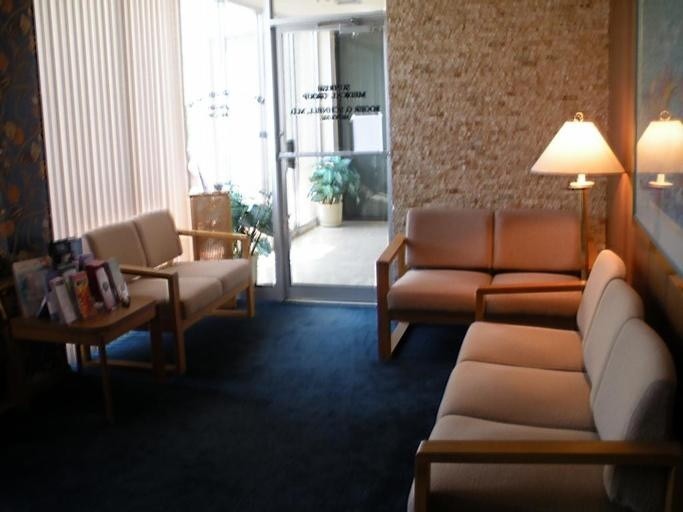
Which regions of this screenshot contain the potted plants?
[307,154,362,227]
[214,178,274,291]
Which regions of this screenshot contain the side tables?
[9,295,166,420]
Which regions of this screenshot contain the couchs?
[81,207,256,374]
[405,247,681,512]
[375,206,583,364]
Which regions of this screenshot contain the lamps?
[635,108,683,242]
[529,111,626,280]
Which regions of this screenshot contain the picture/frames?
[633,1,683,278]
